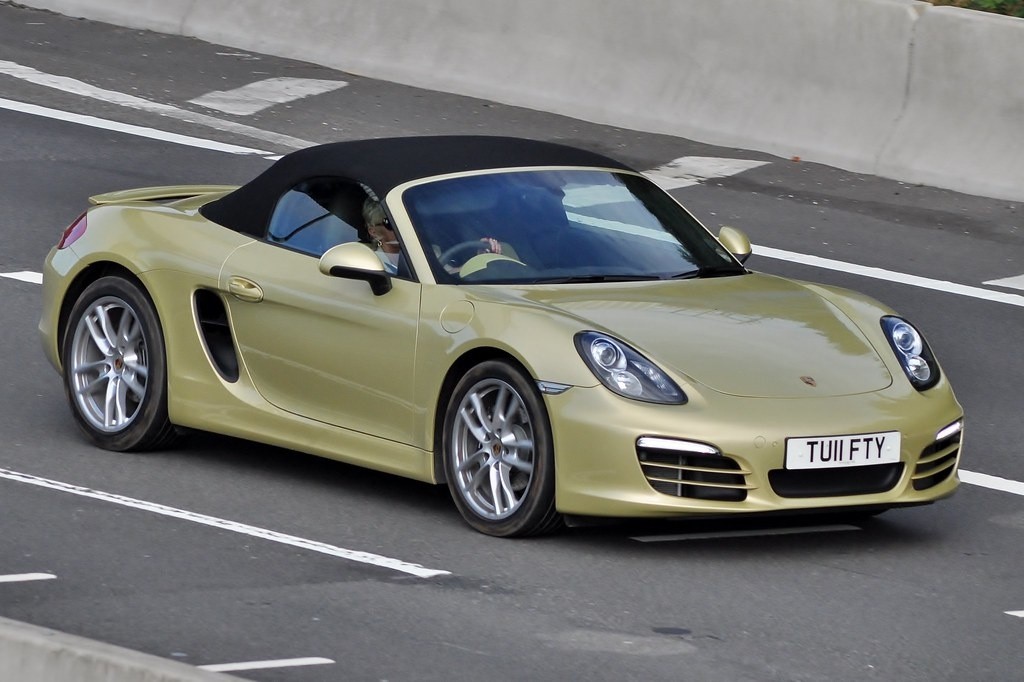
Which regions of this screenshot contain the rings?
[493,240,495,241]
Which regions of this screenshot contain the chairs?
[322,194,368,250]
[455,187,547,268]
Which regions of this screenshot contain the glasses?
[371,217,393,231]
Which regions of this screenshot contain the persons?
[366,197,501,274]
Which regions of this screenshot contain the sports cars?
[38,134,966,540]
